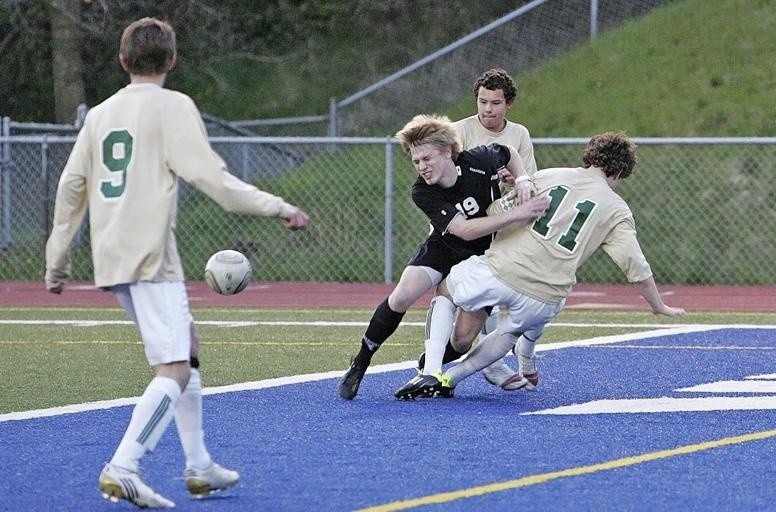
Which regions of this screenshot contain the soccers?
[205,250,251,295]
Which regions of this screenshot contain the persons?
[396,129,689,399]
[337,112,550,400]
[42,16,311,508]
[419,70,545,392]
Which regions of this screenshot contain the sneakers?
[98,463,176,509]
[514,340,540,391]
[481,363,528,391]
[393,367,442,402]
[338,360,366,400]
[419,376,454,398]
[418,352,424,370]
[182,462,239,495]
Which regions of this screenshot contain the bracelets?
[513,172,530,185]
